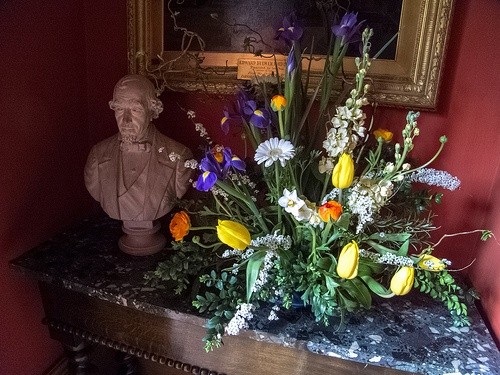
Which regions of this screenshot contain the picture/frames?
[127,0,456,111]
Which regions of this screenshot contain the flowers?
[131,2,498,353]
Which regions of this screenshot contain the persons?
[86,73,196,258]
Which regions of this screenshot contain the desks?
[14,217,500,375]
